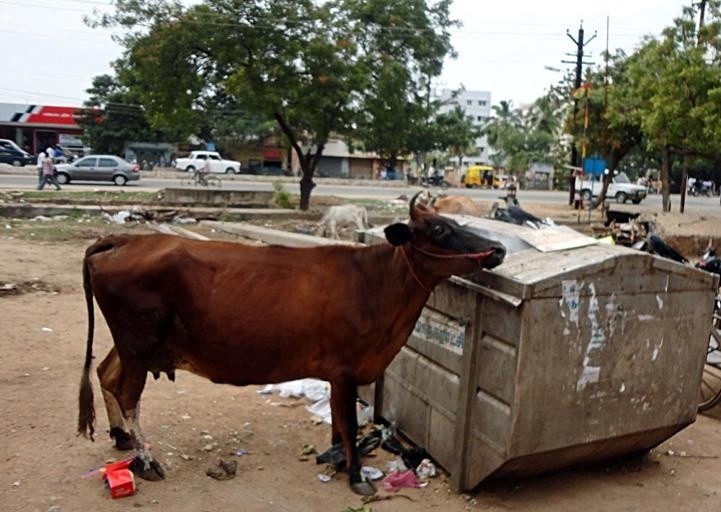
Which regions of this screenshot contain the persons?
[688,176,696,188]
[637,175,645,185]
[46,144,55,157]
[647,175,654,193]
[199,155,209,185]
[484,171,492,184]
[695,177,701,189]
[428,159,440,185]
[54,146,62,163]
[36,149,52,186]
[507,179,516,199]
[37,153,62,191]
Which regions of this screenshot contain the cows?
[74,190,506,497]
[315,203,368,239]
[434,195,481,217]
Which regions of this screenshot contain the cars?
[464,167,518,190]
[175,150,244,175]
[51,154,141,187]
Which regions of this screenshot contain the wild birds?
[637,221,689,264]
[497,194,551,230]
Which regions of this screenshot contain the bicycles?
[177,167,223,190]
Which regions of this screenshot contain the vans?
[0,138,31,168]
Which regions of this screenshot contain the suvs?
[574,168,648,205]
